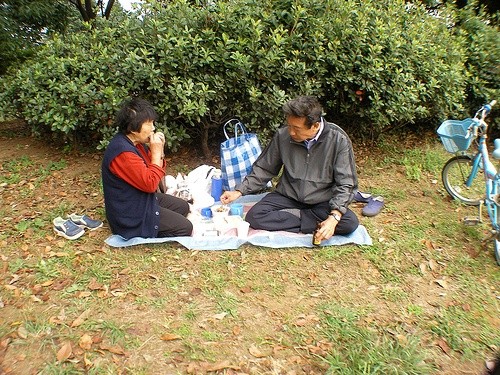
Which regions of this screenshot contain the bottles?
[313,221,322,245]
[211,169,223,201]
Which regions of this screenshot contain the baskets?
[437,118,476,152]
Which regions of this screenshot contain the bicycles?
[436,99,500,266]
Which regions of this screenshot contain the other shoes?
[362,196,385,216]
[353,191,373,203]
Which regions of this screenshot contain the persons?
[218,96,360,242]
[103,98,194,237]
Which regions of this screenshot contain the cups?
[231,203,244,216]
[201,208,211,217]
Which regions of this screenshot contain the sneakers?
[52,217,85,240]
[68,213,103,230]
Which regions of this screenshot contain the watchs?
[328,213,340,222]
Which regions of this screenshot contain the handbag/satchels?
[220,119,272,191]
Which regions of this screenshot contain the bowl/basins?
[211,205,230,218]
